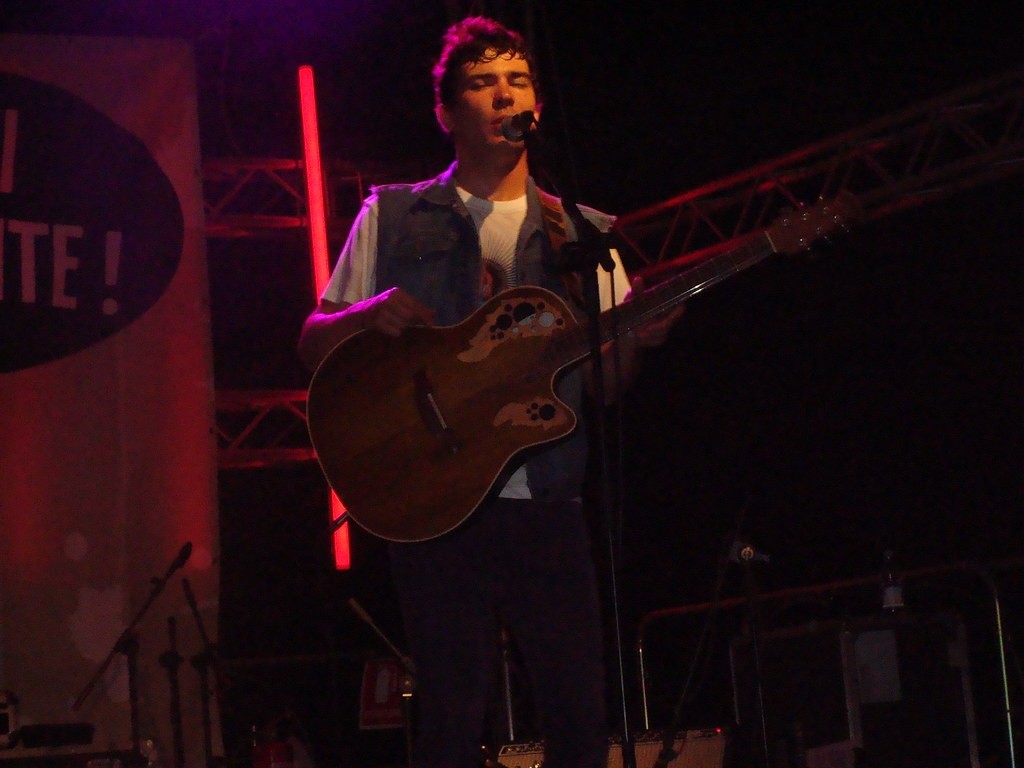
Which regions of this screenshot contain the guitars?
[304,184,867,545]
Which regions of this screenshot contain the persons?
[293,14,687,767]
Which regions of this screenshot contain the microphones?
[501,110,534,143]
[168,541,192,575]
